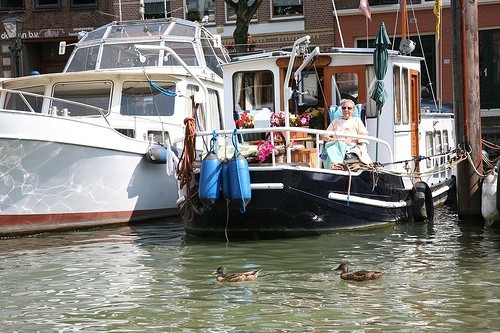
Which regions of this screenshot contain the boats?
[0,0,234,239]
[175,0,459,243]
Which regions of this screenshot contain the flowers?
[270,107,324,127]
[236,111,254,129]
[257,138,305,163]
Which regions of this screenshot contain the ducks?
[210,263,264,283]
[330,262,387,282]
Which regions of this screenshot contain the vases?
[275,126,309,139]
[276,148,317,163]
[237,132,266,143]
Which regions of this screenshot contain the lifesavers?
[412,182,434,222]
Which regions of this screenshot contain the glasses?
[341,106,353,110]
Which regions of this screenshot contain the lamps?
[134,48,146,63]
[201,15,209,26]
[77,31,87,40]
[143,24,152,36]
[399,39,416,56]
[164,53,171,61]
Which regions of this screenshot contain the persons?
[322,98,375,170]
[276,3,296,15]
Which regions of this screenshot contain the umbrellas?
[370,22,391,162]
